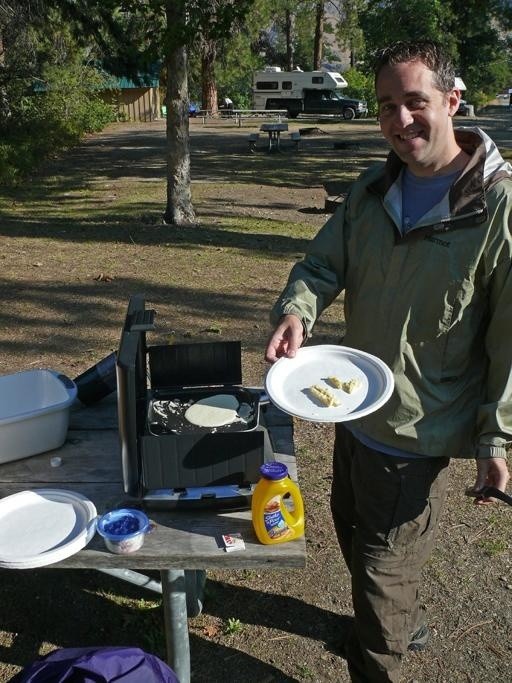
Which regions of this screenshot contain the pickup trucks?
[265,88,368,119]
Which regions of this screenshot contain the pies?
[183,394,239,427]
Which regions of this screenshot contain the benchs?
[248,132,301,158]
[196,114,288,129]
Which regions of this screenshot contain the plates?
[0,487,99,570]
[265,344,396,426]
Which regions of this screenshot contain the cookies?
[327,375,341,388]
[342,376,362,393]
[308,384,342,408]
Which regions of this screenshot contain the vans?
[252,67,349,112]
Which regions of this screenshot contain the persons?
[264,37,512,682]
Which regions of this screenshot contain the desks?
[260,123,288,154]
[0,383,308,683]
[199,109,289,127]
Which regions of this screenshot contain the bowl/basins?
[97,507,150,554]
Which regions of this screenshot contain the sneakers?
[408,626,429,650]
